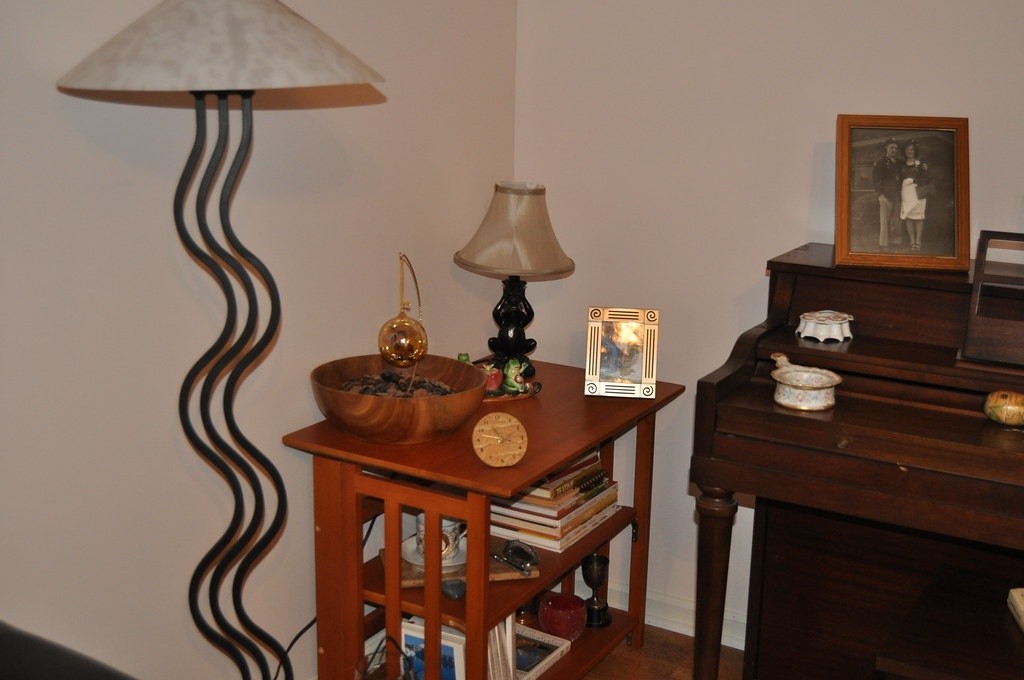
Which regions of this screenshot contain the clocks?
[472,412,528,467]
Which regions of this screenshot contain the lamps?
[57,0,387,680]
[454,183,575,380]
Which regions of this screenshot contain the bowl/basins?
[311,354,488,443]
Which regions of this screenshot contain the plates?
[401,535,467,573]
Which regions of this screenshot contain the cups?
[416,513,467,559]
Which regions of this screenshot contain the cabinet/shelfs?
[283,351,685,680]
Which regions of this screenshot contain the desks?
[686,243,1024,680]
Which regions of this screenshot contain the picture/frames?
[836,113,972,272]
[584,305,661,400]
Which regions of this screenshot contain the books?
[489,452,622,554]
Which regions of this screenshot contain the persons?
[873,137,935,250]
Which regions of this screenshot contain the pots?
[770,353,842,409]
